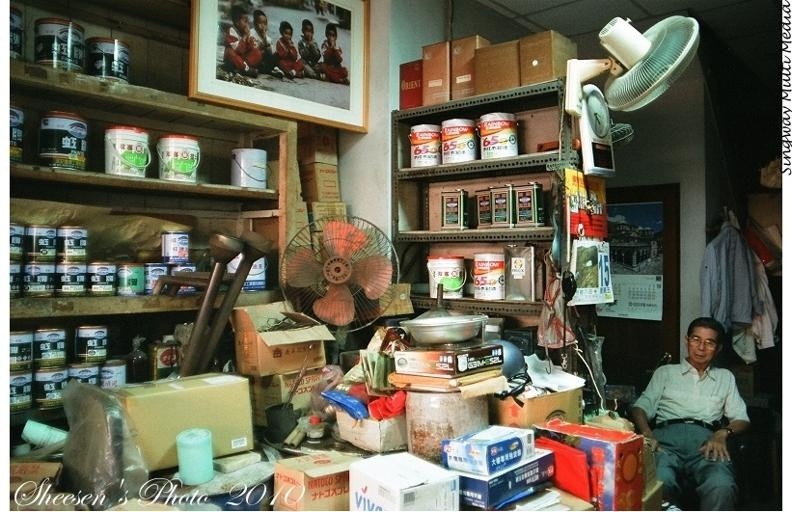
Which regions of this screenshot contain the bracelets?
[720,427,734,438]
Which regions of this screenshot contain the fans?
[277,212,401,356]
[607,116,634,150]
[564,14,701,121]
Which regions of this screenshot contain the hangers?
[722,204,730,227]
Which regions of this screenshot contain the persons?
[223,5,350,85]
[631,317,752,511]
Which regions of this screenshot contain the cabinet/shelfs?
[10,0,290,424]
[394,82,566,330]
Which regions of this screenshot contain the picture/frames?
[187,0,371,136]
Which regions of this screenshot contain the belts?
[655,418,715,432]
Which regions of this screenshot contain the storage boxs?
[358,284,414,320]
[490,392,585,434]
[348,450,460,512]
[397,30,579,114]
[643,482,665,511]
[98,372,257,475]
[229,301,335,377]
[242,363,344,427]
[462,447,557,510]
[644,437,659,493]
[441,425,534,477]
[336,405,410,456]
[288,129,380,265]
[275,452,364,510]
[10,457,66,510]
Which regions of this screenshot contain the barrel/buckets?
[408,124,442,168]
[471,254,507,300]
[34,16,85,74]
[426,255,468,299]
[441,118,478,164]
[229,147,272,190]
[38,109,89,172]
[240,253,268,292]
[155,133,200,183]
[104,124,152,178]
[85,36,130,84]
[10,7,23,60]
[479,111,519,159]
[10,105,26,162]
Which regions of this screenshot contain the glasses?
[690,337,717,351]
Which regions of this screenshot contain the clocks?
[572,82,617,178]
